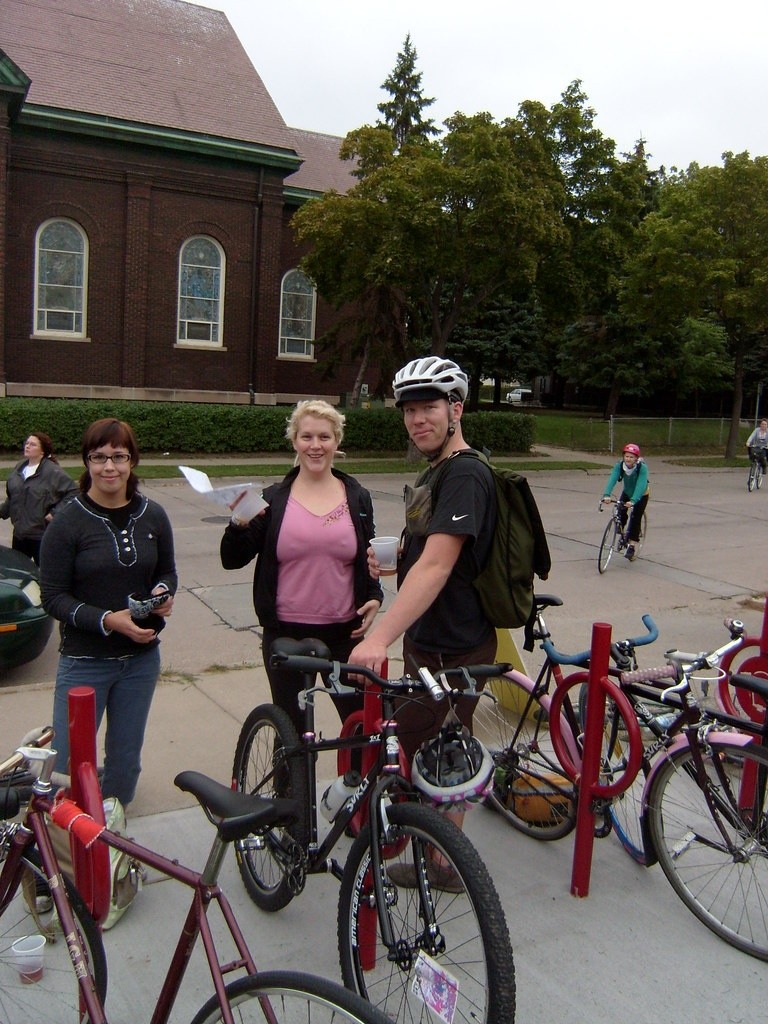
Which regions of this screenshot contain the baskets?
[748,447,762,459]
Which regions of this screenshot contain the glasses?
[24,441,42,447]
[87,454,132,464]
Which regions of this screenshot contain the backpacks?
[412,449,552,628]
[23,798,146,941]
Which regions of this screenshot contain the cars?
[506,389,532,403]
[0,545,55,672]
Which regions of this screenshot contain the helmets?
[410,735,497,812]
[391,356,468,400]
[624,444,640,458]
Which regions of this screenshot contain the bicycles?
[0,724,395,1024]
[597,500,647,574]
[747,445,768,492]
[469,593,768,963]
[232,652,516,1024]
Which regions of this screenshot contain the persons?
[602,442,650,558]
[349,357,499,895]
[745,418,768,484]
[0,431,78,568]
[220,399,385,869]
[23,418,178,915]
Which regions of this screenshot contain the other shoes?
[385,844,465,893]
[624,545,635,560]
[616,520,624,534]
[762,467,766,475]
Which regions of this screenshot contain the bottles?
[320,769,362,821]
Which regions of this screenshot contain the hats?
[396,389,461,408]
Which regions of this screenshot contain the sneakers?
[23,869,54,914]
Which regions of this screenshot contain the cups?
[368,537,399,576]
[12,935,47,985]
[231,490,269,526]
